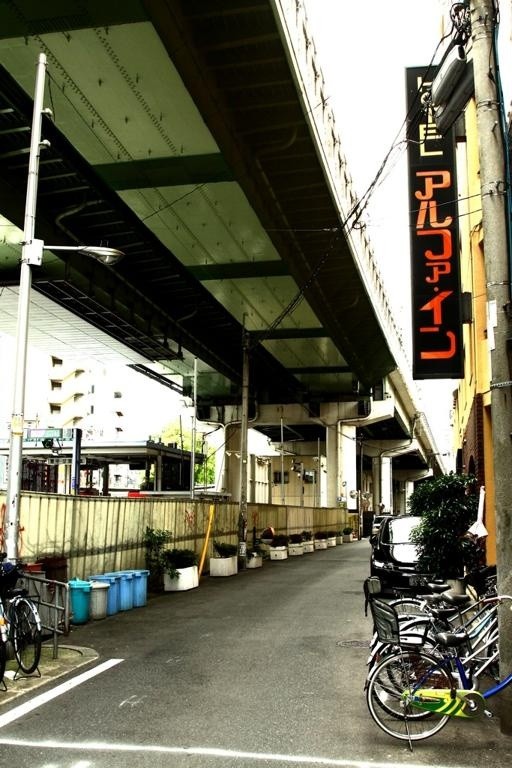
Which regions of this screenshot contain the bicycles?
[0,606,9,693]
[363,560,511,752]
[0,551,44,682]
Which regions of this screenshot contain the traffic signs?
[30,428,64,438]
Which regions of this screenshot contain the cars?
[370,516,394,535]
[370,515,432,595]
[397,512,410,518]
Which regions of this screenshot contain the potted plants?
[405,468,483,605]
[133,520,355,596]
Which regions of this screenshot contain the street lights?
[4,231,126,565]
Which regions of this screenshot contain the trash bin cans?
[68,570,147,623]
